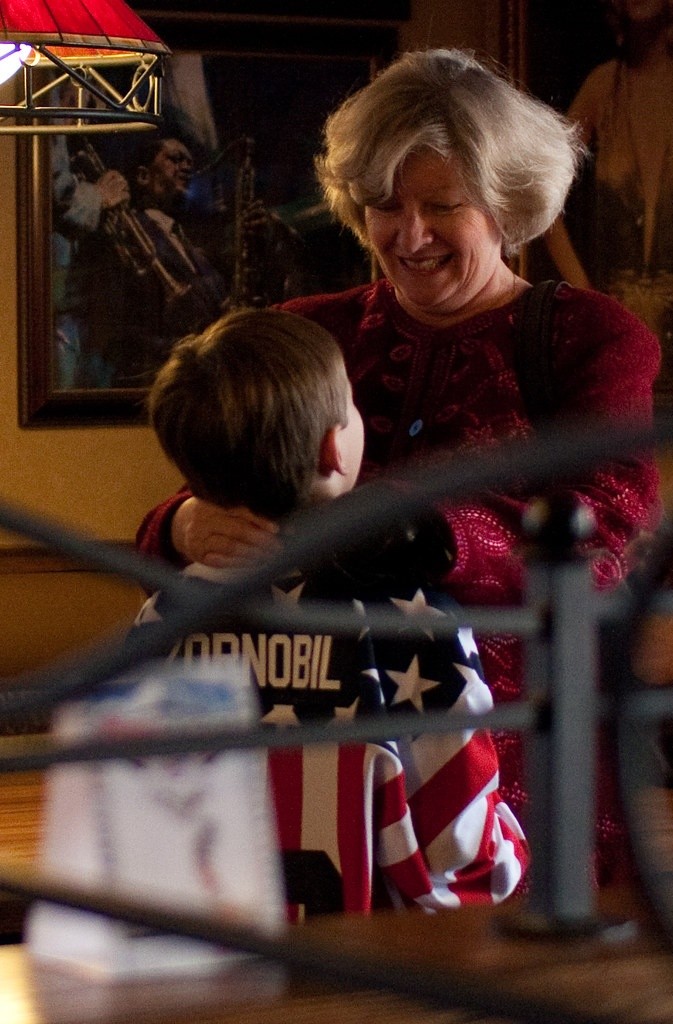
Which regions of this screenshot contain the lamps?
[0,0,174,136]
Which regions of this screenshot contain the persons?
[135,50,664,904]
[124,308,533,924]
[544,0,673,290]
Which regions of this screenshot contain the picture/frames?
[16,13,399,433]
[500,0,673,407]
